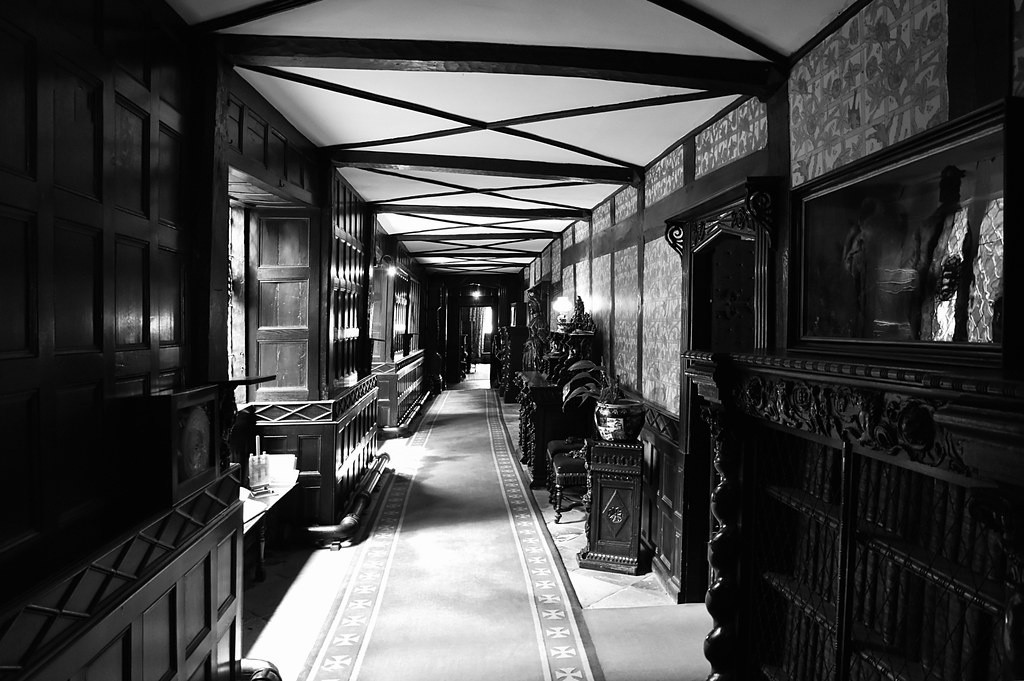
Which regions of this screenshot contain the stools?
[546,438,595,524]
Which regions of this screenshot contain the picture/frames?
[787,96,1024,367]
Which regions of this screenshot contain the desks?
[516,369,601,490]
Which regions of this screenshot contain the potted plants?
[562,359,648,442]
